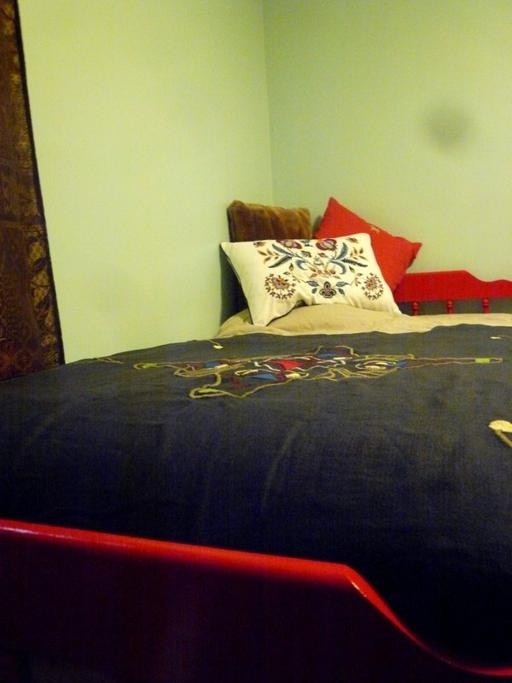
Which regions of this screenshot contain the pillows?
[217,194,424,333]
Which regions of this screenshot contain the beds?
[0,268,512,683]
